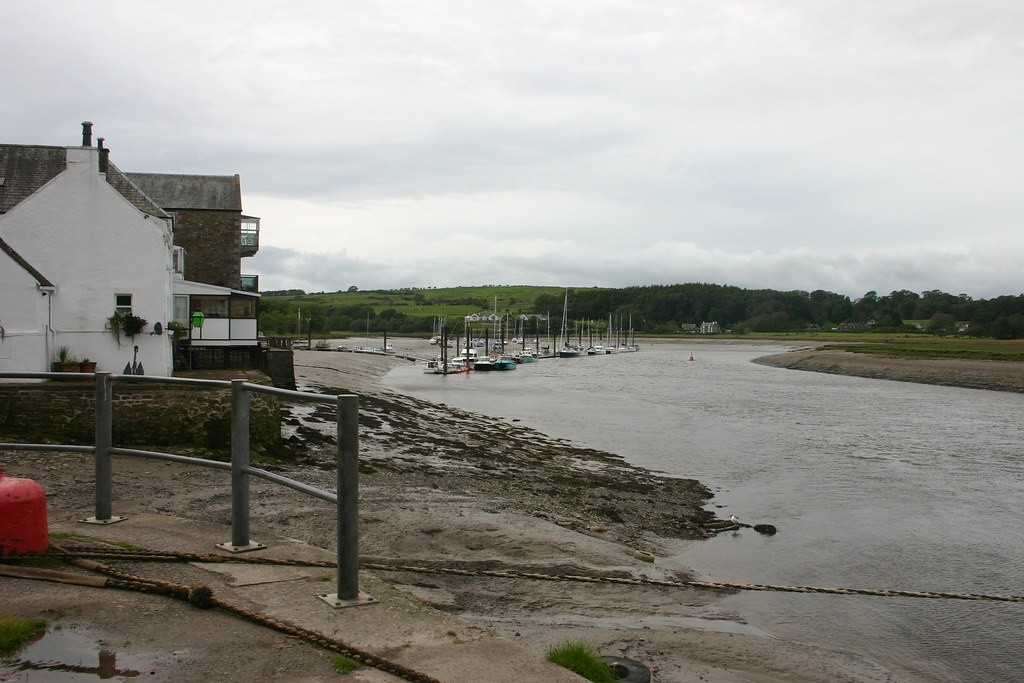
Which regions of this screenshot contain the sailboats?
[418,290,641,375]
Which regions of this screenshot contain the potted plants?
[52,345,98,381]
